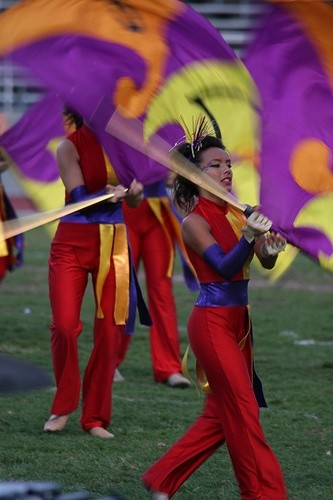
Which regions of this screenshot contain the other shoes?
[167,373,191,388]
[147,484,169,500]
[89,427,114,440]
[114,371,124,382]
[44,415,68,431]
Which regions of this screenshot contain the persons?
[139,134,289,500]
[44,102,145,438]
[113,181,191,388]
